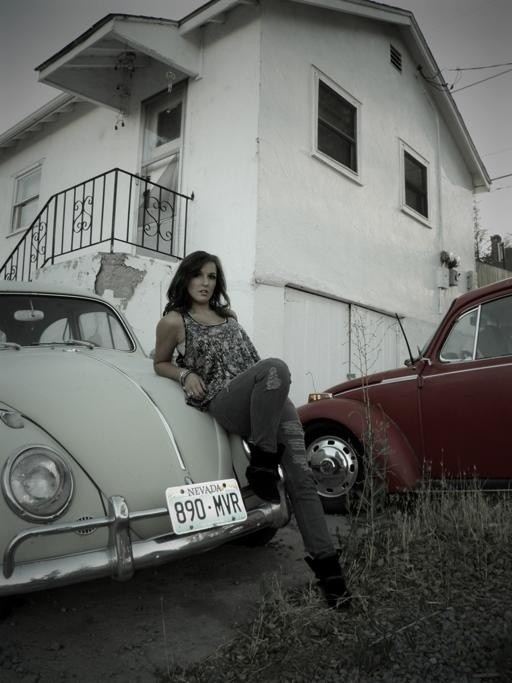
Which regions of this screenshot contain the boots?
[245,440,285,504]
[304,549,352,611]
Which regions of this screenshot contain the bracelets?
[178,368,193,387]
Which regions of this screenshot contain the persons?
[151,249,362,614]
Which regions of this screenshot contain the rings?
[188,391,193,397]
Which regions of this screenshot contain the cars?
[0,275,301,617]
[291,275,512,518]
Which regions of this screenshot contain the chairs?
[476,325,509,358]
